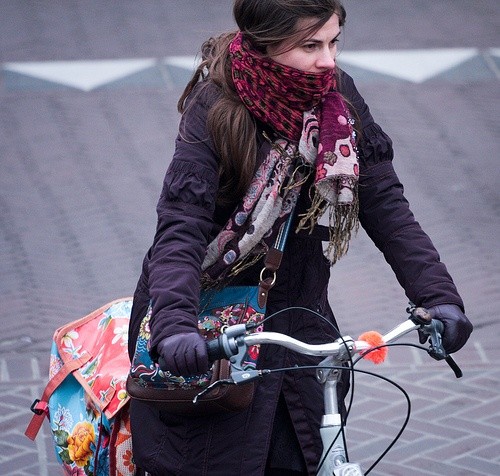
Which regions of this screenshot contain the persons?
[127,0,473,476]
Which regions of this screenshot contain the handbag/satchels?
[125,284,268,414]
[26,297,138,476]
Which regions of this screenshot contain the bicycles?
[158,306,462,476]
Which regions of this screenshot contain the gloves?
[417,304,473,360]
[157,332,208,378]
